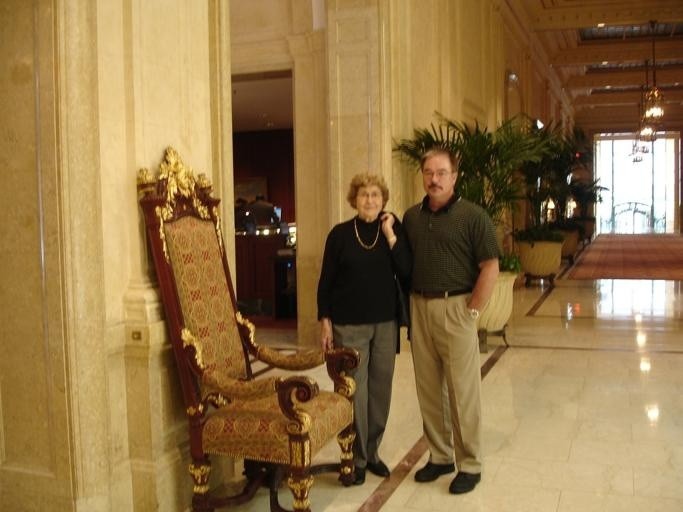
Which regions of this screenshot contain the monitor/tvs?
[270,206,282,225]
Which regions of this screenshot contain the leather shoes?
[448,472,480,494]
[352,465,365,485]
[414,463,454,483]
[367,461,390,477]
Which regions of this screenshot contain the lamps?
[624,18,667,164]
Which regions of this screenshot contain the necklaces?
[352,218,381,250]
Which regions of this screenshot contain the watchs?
[467,308,480,319]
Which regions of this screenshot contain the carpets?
[569,232,682,280]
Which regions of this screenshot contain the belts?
[411,289,472,298]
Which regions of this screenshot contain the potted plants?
[390,109,610,333]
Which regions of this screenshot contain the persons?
[248,193,279,226]
[399,146,503,494]
[234,197,255,233]
[314,169,414,482]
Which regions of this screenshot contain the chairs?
[139,145,361,512]
